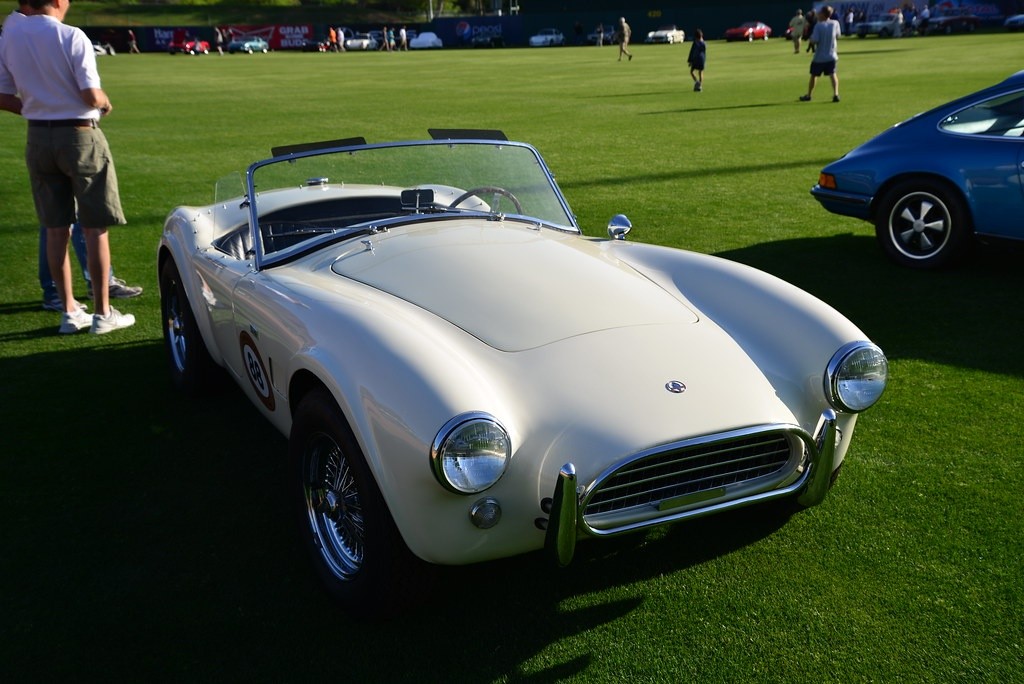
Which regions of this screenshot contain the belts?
[27,116,99,128]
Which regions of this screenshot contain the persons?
[798,5,842,101]
[596,23,604,47]
[0,0,136,336]
[0,0,144,307]
[688,29,706,92]
[789,7,866,54]
[329,27,345,52]
[128,29,139,55]
[379,25,408,52]
[617,17,633,62]
[215,27,234,56]
[892,3,930,38]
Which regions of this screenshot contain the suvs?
[587,24,620,45]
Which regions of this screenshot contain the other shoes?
[799,95,811,100]
[628,55,632,61]
[833,96,840,102]
[694,87,701,92]
[694,81,700,90]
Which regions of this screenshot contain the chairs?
[219,221,323,259]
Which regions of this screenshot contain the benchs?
[254,197,443,231]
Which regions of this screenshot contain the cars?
[646,24,685,44]
[1004,13,1024,29]
[226,36,269,54]
[410,32,444,49]
[157,126,889,597]
[928,9,980,34]
[301,27,417,52]
[811,70,1024,272]
[786,26,810,40]
[472,33,506,48]
[529,28,566,47]
[167,36,210,54]
[854,13,918,39]
[724,22,772,42]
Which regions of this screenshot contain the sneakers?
[41,294,88,311]
[59,308,94,333]
[109,281,143,298]
[90,305,135,335]
